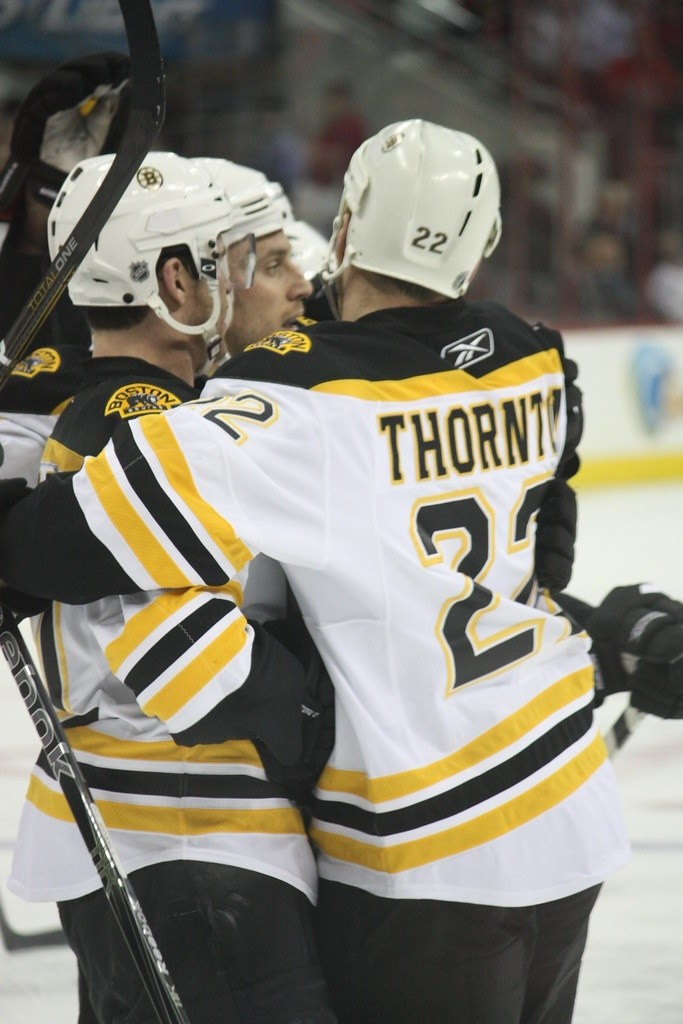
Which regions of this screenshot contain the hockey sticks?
[0,0,174,396]
[0,609,190,1024]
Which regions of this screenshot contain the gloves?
[582,582,683,720]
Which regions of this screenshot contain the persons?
[0,47,683,1024]
[321,80,368,155]
[250,87,302,186]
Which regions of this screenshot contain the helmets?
[0,51,131,220]
[269,182,306,255]
[47,152,233,335]
[189,156,284,279]
[289,222,340,279]
[323,119,503,300]
